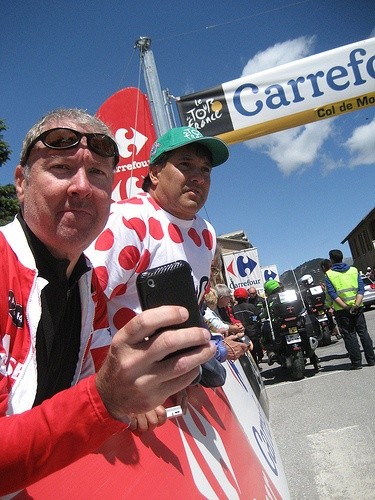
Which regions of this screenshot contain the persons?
[298,259,342,339]
[200,283,269,371]
[0,108,217,500]
[324,250,375,370]
[84,127,229,432]
[365,266,375,278]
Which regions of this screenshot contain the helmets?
[234,288,247,299]
[265,280,280,295]
[301,274,314,286]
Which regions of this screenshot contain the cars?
[358,274,375,307]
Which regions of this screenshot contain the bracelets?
[221,340,235,363]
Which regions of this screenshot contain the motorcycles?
[306,286,344,346]
[257,290,319,380]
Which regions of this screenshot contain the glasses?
[23,127,119,168]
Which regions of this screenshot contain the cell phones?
[165,404,183,419]
[135,260,203,363]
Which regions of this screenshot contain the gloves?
[344,305,359,317]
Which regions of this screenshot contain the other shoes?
[348,365,362,370]
[257,357,262,363]
[314,363,321,372]
[267,352,275,366]
[336,335,343,339]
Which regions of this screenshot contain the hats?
[149,126,229,167]
